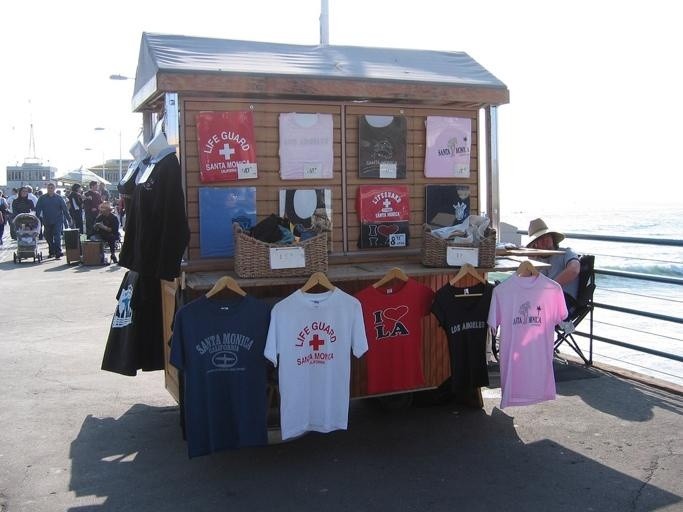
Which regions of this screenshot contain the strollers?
[12,212,43,264]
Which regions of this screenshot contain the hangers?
[372,266,410,289]
[510,260,539,281]
[204,274,247,306]
[295,270,336,296]
[451,263,488,289]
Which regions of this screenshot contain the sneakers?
[48,253,61,259]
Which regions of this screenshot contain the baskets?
[233,221,329,278]
[421,223,496,268]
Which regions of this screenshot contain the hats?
[524,218,564,248]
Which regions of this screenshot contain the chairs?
[552,256,595,368]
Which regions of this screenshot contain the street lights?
[84,147,105,180]
[94,127,122,180]
[109,74,135,81]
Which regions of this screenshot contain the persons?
[116,141,151,233]
[118,131,190,282]
[0,180,125,264]
[523,218,581,313]
